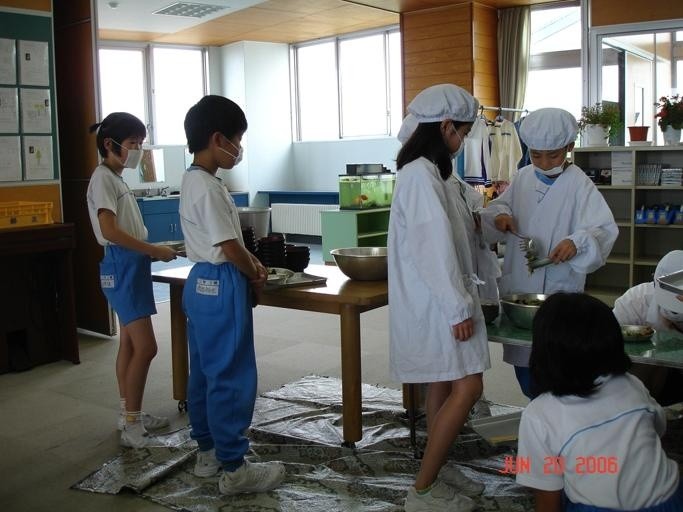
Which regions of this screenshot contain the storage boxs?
[0,199,54,231]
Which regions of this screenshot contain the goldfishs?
[353,197,366,204]
[361,195,368,200]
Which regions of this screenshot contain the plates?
[526,256,549,270]
[147,239,186,258]
[628,141,653,146]
[621,324,656,343]
[261,267,294,284]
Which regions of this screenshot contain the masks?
[660,307,683,322]
[216,135,243,165]
[104,136,143,169]
[444,124,465,160]
[528,144,569,177]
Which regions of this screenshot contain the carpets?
[65,372,533,511]
[151,255,196,305]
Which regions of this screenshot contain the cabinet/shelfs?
[319,208,391,265]
[572,146,683,308]
[135,198,143,217]
[143,197,186,243]
[230,192,249,207]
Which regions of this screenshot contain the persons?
[87,112,181,451]
[387,83,492,512]
[612,249,683,334]
[399,114,502,423]
[514,289,683,512]
[480,108,619,399]
[179,94,286,494]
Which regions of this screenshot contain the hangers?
[478,106,530,128]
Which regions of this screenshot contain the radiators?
[271,203,340,236]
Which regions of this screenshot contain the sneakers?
[193,448,220,478]
[404,479,476,511]
[436,462,486,497]
[117,411,170,431]
[219,458,286,497]
[119,428,165,450]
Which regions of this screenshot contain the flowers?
[654,94,683,131]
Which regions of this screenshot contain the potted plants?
[576,103,623,148]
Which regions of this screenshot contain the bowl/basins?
[499,292,549,329]
[479,303,499,325]
[626,126,650,141]
[238,223,309,272]
[330,248,389,281]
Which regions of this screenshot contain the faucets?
[160,186,170,195]
[147,188,151,195]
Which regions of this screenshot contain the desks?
[1,220,81,379]
[486,314,682,374]
[151,263,388,449]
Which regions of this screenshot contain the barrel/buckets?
[580,123,609,147]
[237,207,272,240]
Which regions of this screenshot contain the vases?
[661,125,681,144]
[628,126,650,142]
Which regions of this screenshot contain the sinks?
[142,195,181,201]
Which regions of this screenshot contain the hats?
[518,108,579,150]
[656,250,683,315]
[407,83,480,124]
